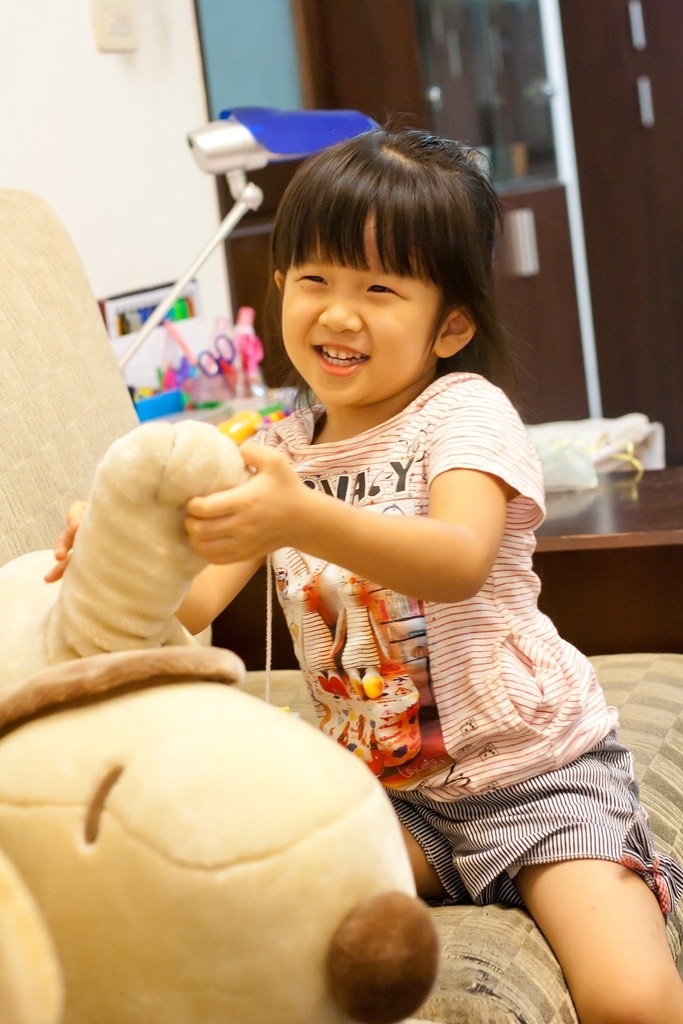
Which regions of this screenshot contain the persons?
[42,114,683,1024]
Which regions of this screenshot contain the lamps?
[119,105,383,380]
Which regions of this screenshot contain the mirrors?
[191,1,558,206]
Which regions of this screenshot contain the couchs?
[1,182,683,1024]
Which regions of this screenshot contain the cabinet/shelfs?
[190,0,683,473]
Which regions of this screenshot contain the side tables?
[212,467,682,671]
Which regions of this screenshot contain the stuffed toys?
[0,416,440,1024]
